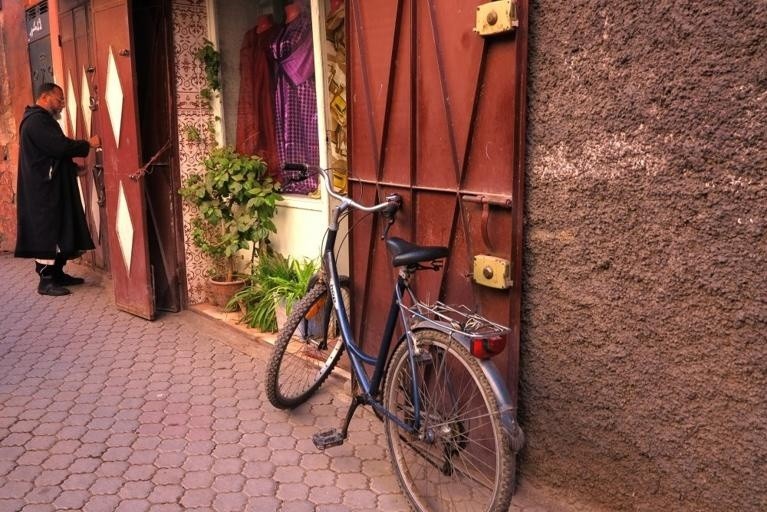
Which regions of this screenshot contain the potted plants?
[177,147,325,339]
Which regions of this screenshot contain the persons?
[10,82,103,296]
[236,12,282,190]
[269,1,320,195]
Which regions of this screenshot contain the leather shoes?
[38,274,84,295]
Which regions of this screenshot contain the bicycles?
[265,162,524,512]
